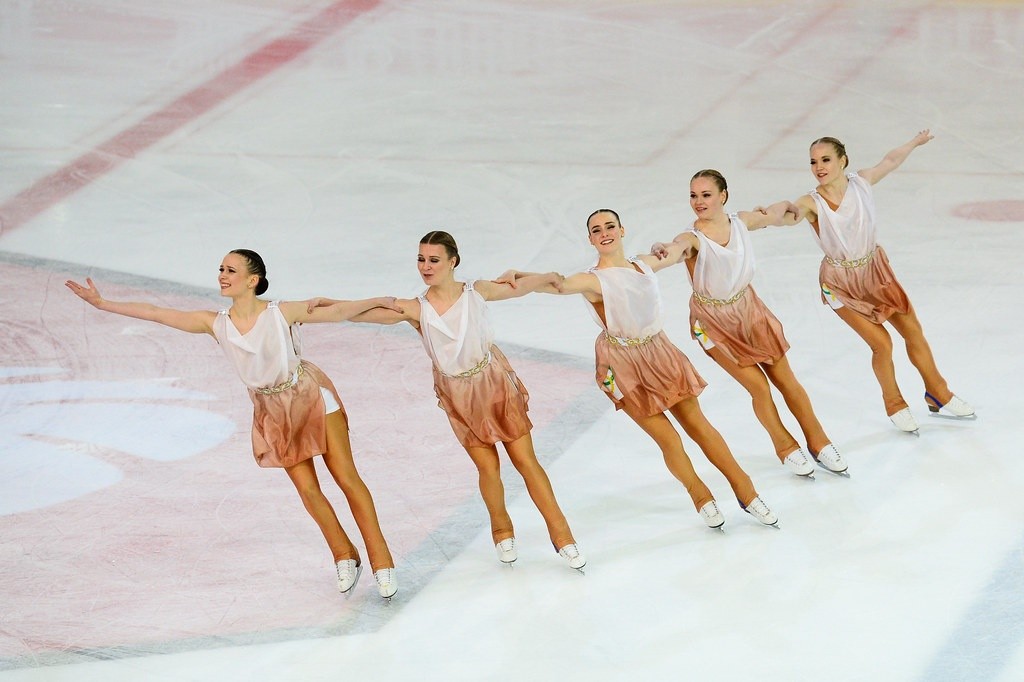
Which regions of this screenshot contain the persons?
[64,249,404,598]
[750,128,976,437]
[293,230,588,573]
[490,209,782,534]
[647,169,848,480]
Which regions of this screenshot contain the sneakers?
[336,559,364,598]
[784,449,817,480]
[699,499,725,533]
[560,543,587,574]
[745,496,779,528]
[891,407,922,436]
[928,393,976,418]
[374,568,397,604]
[808,442,848,476]
[497,537,517,568]
